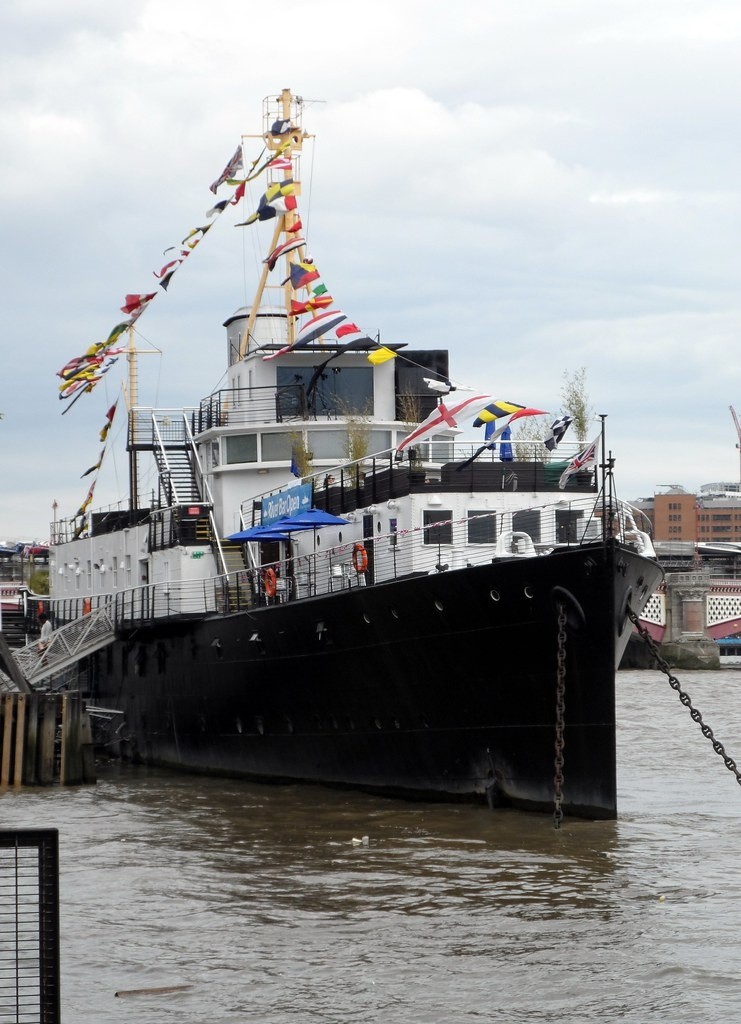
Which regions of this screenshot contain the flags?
[336,323,361,339]
[558,436,599,488]
[290,262,321,289]
[367,347,398,365]
[210,145,243,195]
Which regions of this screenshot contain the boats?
[50,90,665,822]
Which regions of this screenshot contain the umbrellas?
[222,508,350,603]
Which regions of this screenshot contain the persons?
[38,613,52,667]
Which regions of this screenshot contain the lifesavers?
[82,598,91,617]
[264,567,277,596]
[351,544,368,574]
[37,601,43,616]
